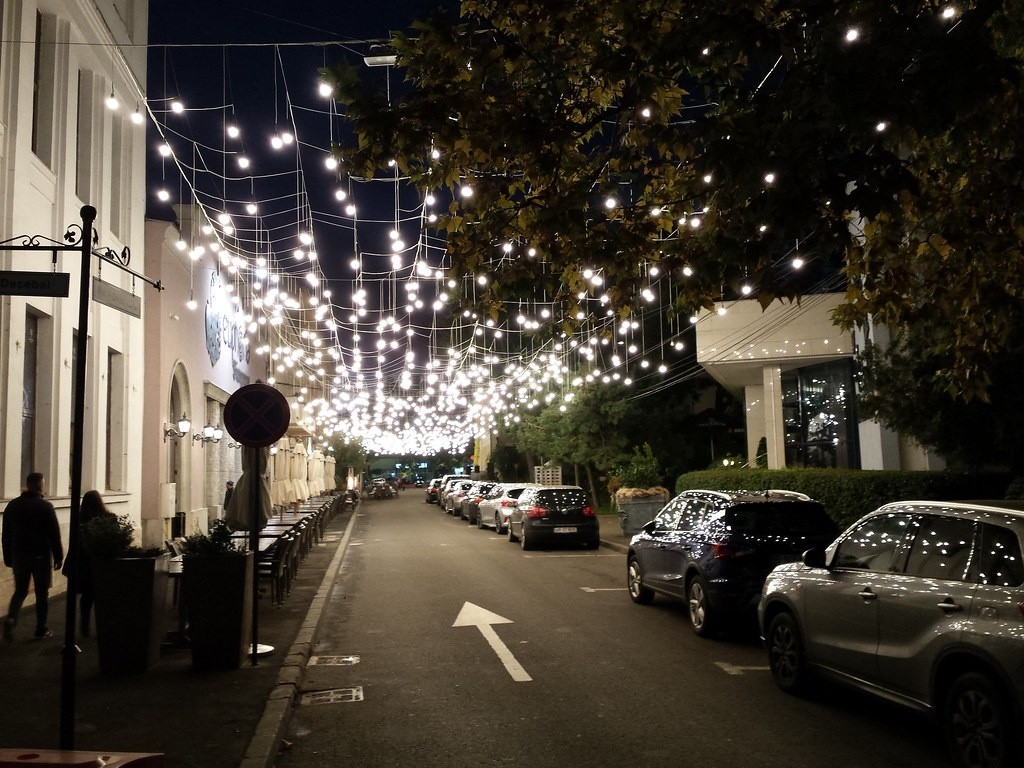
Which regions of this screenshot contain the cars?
[507,485,600,550]
[441,479,472,509]
[460,482,499,524]
[425,479,442,503]
[446,481,481,516]
[476,482,543,534]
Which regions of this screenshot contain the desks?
[274,512,312,519]
[267,518,302,527]
[229,531,286,538]
[262,526,294,533]
[170,538,278,602]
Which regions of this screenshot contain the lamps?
[163,412,191,443]
[193,421,224,448]
[228,441,243,449]
[269,444,278,456]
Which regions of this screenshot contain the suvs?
[759,500,1024,766]
[436,475,471,505]
[624,489,843,638]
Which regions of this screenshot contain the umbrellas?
[221,434,336,550]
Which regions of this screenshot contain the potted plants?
[179,518,255,671]
[80,513,166,677]
[608,441,670,538]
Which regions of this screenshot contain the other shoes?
[34,628,54,639]
[4,618,18,631]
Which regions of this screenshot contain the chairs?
[164,488,358,609]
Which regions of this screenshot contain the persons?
[2,470,64,639]
[438,474,443,479]
[224,480,235,510]
[493,471,499,482]
[392,476,407,496]
[62,490,123,637]
[347,473,360,499]
